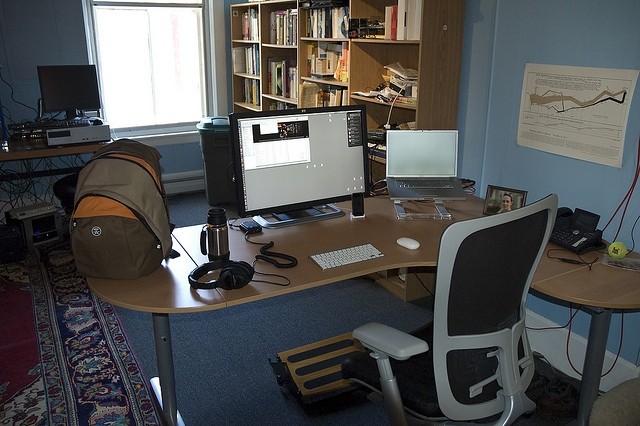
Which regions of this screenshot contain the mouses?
[396,236,421,250]
[88,116,102,126]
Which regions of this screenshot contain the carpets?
[0,235,166,425]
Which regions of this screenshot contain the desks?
[0,132,116,185]
[85,193,640,425]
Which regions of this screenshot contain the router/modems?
[239,220,263,235]
[9,203,58,220]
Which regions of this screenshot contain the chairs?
[340,191,559,426]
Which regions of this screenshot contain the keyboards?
[311,243,385,270]
[9,117,90,134]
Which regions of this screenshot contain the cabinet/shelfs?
[229,0,297,114]
[297,0,467,304]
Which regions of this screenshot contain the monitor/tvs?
[37,65,103,125]
[228,104,371,229]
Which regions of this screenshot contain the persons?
[496,193,514,213]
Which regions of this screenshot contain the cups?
[200,207,230,263]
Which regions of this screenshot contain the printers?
[20,212,64,250]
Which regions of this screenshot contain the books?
[267,57,297,98]
[305,6,349,38]
[269,9,297,46]
[298,83,348,109]
[385,0,422,41]
[241,8,259,41]
[242,79,260,106]
[232,44,260,76]
[307,41,350,83]
[269,102,297,111]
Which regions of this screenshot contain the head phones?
[188,258,255,291]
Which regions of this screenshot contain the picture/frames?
[481,183,529,225]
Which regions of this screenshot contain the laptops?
[385,128,467,201]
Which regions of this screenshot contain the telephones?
[549,207,607,254]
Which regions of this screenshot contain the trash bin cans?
[195,114,241,205]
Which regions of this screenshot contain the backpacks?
[69,137,179,280]
